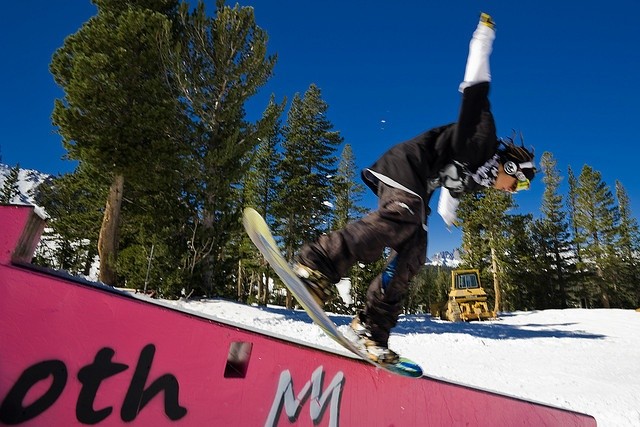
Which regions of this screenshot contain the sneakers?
[348,316,401,367]
[294,260,336,308]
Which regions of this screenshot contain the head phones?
[504,160,536,174]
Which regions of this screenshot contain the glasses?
[513,175,532,195]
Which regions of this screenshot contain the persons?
[289,11,537,362]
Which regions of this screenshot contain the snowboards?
[241,206,424,379]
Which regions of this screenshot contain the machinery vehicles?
[440,269,489,321]
